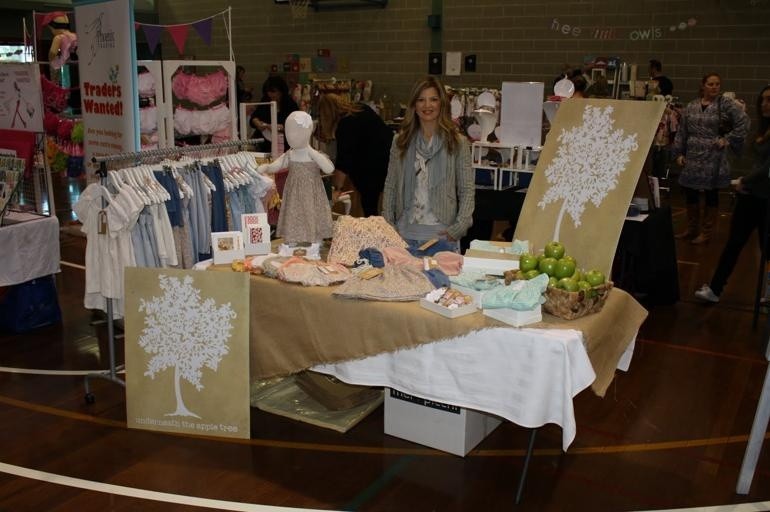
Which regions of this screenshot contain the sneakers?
[694,286,718,302]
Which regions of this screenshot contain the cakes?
[435,288,472,309]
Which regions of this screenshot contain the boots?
[674,205,701,240]
[690,206,718,245]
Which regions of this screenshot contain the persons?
[552,68,587,99]
[256,111,338,247]
[692,87,769,305]
[236,66,253,132]
[646,59,676,96]
[670,73,752,245]
[249,76,301,154]
[382,75,475,255]
[315,92,393,216]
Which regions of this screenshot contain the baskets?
[503,269,614,320]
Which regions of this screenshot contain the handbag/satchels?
[719,122,744,157]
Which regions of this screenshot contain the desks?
[194,238,629,506]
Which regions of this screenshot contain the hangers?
[79,138,273,225]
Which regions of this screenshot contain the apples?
[514,241,604,292]
